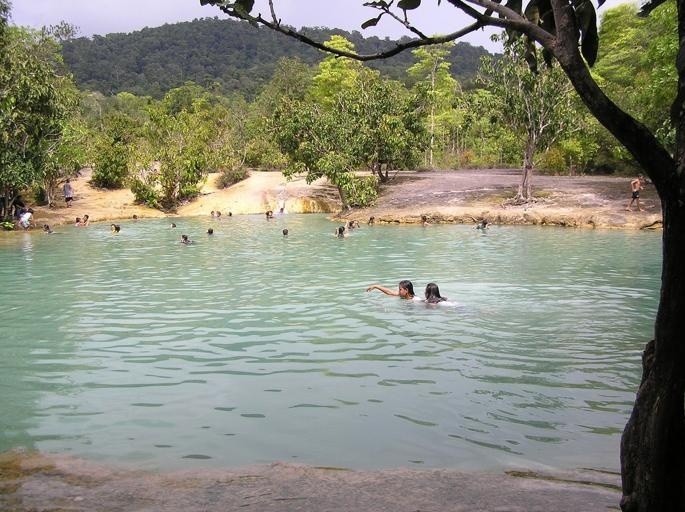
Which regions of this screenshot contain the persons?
[366,280,420,302]
[63,179,73,208]
[13,196,491,244]
[424,283,447,304]
[624,173,644,212]
